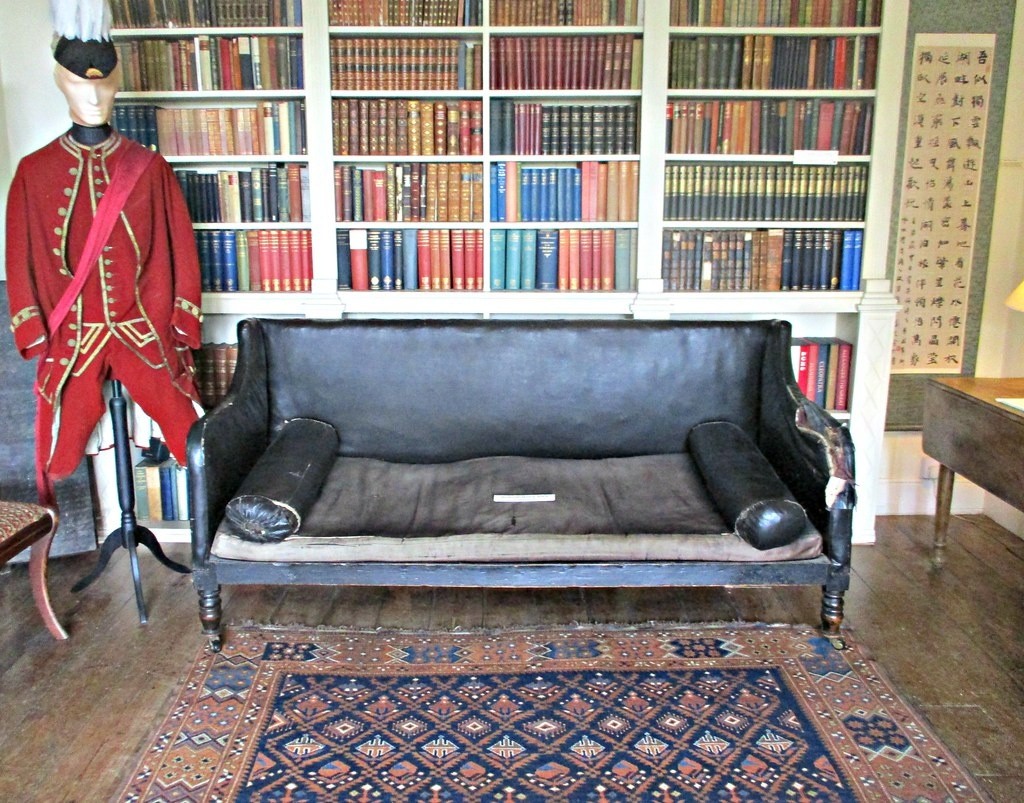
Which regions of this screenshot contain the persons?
[6,1,202,517]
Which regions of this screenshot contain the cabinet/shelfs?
[90,0,912,544]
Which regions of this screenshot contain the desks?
[921,378,1024,570]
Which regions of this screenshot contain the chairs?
[0,499,69,642]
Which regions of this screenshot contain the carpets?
[112,618,998,803]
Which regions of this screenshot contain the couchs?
[187,318,858,652]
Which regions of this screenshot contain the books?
[103,1,884,522]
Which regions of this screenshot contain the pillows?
[226,418,339,546]
[689,421,808,551]
